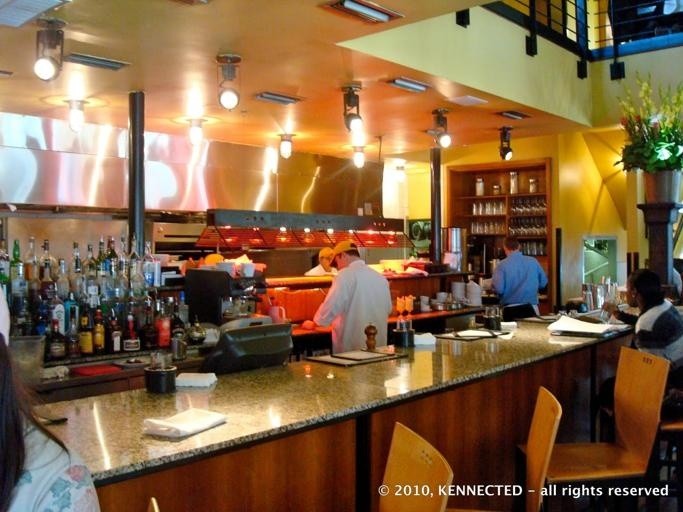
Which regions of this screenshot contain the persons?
[601,268,683,421]
[0,334,100,512]
[315,240,393,354]
[304,247,338,276]
[493,235,549,322]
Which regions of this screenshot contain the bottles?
[1,232,210,360]
[580,281,619,311]
[469,171,548,258]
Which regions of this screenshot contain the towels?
[176,372,217,387]
[413,332,437,346]
[501,321,517,330]
[142,409,227,440]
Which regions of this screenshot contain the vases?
[641,169,681,203]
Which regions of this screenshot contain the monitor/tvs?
[204,323,294,374]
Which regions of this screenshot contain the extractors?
[0,204,205,225]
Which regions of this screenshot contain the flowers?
[612,74,683,170]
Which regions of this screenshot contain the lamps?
[215,55,241,112]
[498,126,513,161]
[351,146,366,168]
[277,133,296,159]
[31,15,64,80]
[341,86,363,135]
[432,108,451,148]
[65,100,87,132]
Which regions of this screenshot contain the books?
[547,314,631,338]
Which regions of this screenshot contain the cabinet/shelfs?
[445,158,550,297]
[262,267,484,338]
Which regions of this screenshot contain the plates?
[420,279,481,312]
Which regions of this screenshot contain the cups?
[482,302,508,333]
[143,364,182,396]
[394,292,416,351]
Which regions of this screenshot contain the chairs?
[378,421,454,512]
[517,344,671,512]
[147,497,160,512]
[607,377,682,505]
[524,386,562,511]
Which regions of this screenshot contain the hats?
[329,241,357,268]
[318,247,334,259]
